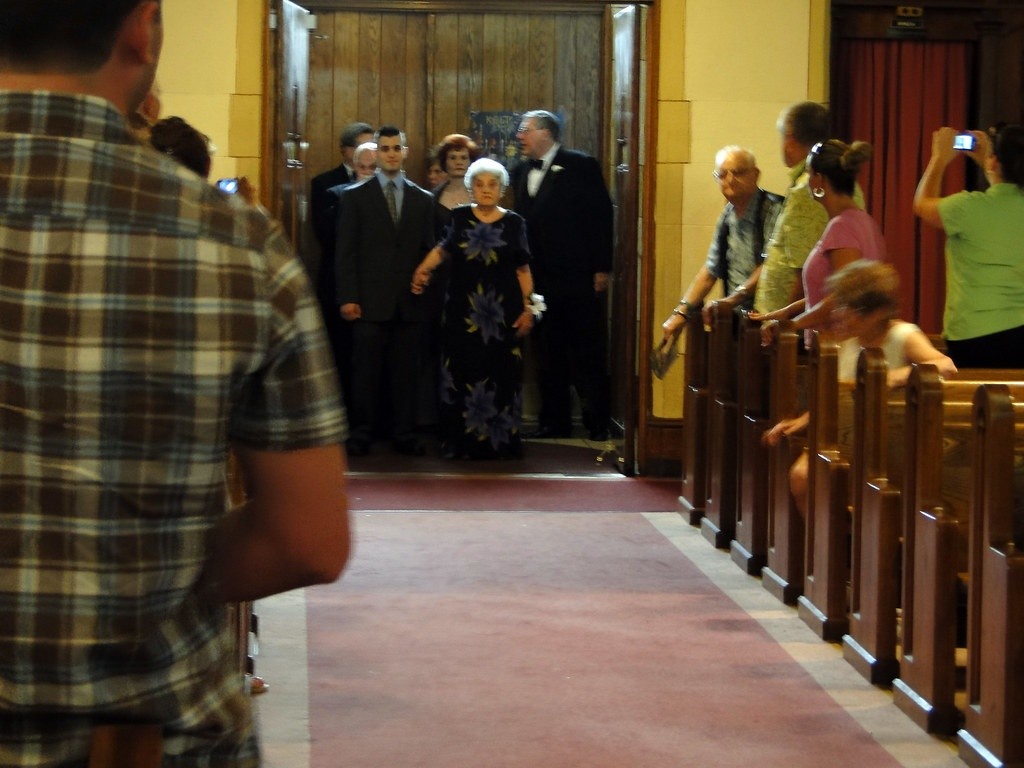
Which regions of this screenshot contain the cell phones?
[952,135,974,151]
[218,179,238,194]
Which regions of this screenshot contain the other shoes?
[519,424,573,440]
[245,673,269,695]
[346,439,372,459]
[392,438,426,456]
[589,425,609,442]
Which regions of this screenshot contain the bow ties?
[528,159,543,169]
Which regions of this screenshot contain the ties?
[384,181,398,226]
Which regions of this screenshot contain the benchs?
[679,299,1024,768]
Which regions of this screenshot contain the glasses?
[517,126,546,134]
[713,167,753,178]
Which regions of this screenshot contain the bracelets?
[679,300,692,313]
[735,286,749,298]
[673,308,689,322]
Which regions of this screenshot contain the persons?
[914,123,1024,370]
[0,0,350,768]
[760,140,886,359]
[766,260,957,525]
[134,81,612,691]
[703,100,866,324]
[661,146,786,347]
